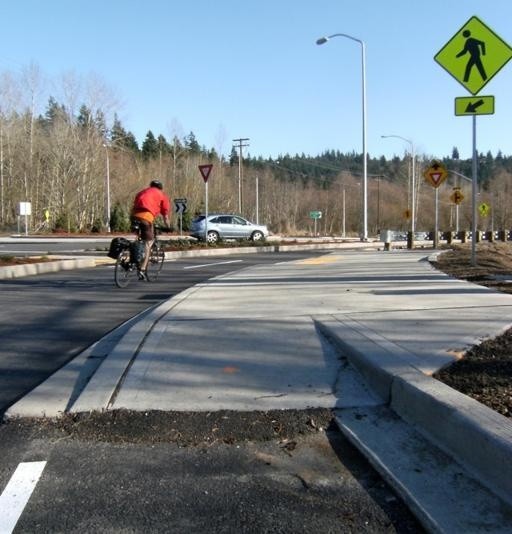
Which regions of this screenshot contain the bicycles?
[115,218,165,289]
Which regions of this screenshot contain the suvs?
[191,213,269,245]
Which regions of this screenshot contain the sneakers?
[137,269,149,282]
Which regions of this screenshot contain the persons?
[130,180,171,282]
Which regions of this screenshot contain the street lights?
[380,135,415,237]
[316,30,369,242]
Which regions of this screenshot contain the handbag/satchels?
[107,237,134,272]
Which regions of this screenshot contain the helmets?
[151,180,162,190]
